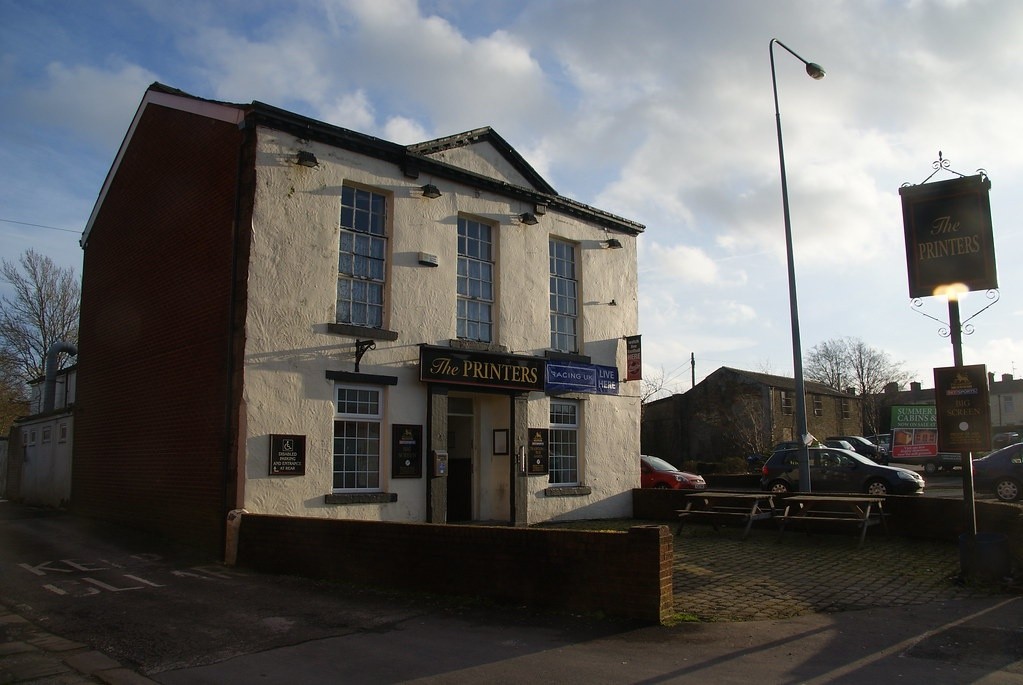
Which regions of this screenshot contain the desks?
[675,492,776,540]
[776,496,891,549]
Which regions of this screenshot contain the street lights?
[768,38,830,492]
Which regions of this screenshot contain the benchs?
[673,509,760,519]
[773,515,867,523]
[712,507,783,513]
[807,510,890,518]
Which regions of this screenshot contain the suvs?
[759,446,926,514]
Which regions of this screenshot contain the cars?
[972,442,1023,504]
[638,453,707,502]
[746,432,890,477]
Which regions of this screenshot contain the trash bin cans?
[959,532,1010,577]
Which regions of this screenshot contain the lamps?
[420,184,442,198]
[515,446,525,472]
[606,239,624,250]
[519,212,540,225]
[296,150,319,167]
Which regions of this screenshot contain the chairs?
[823,454,836,466]
